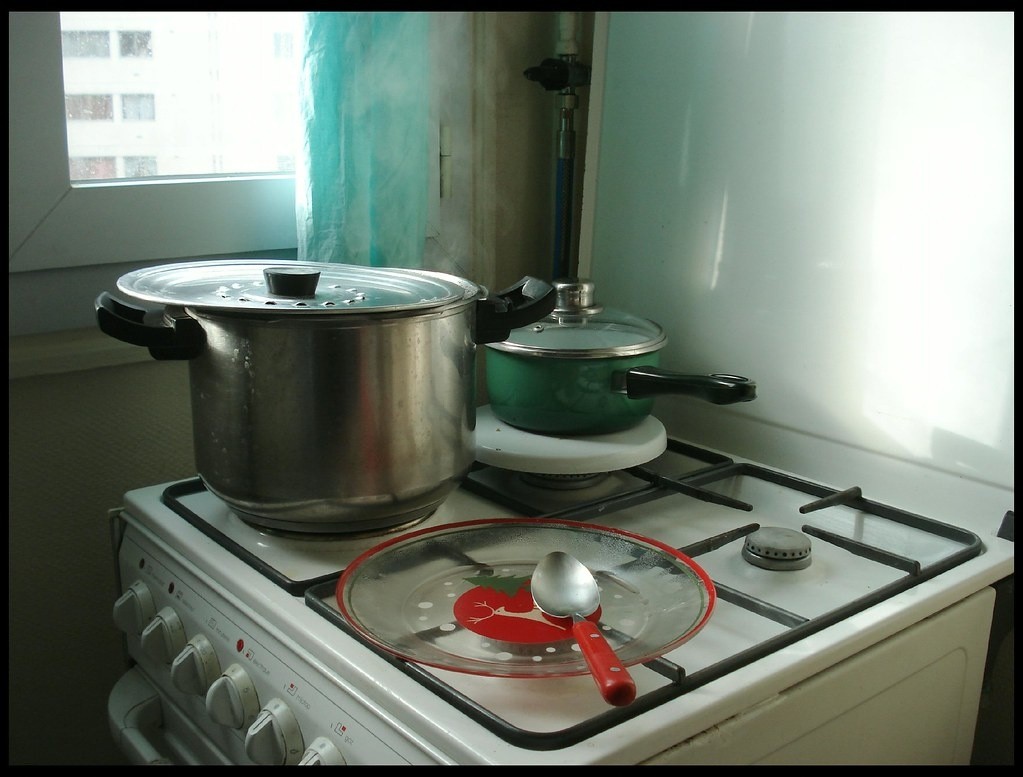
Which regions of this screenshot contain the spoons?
[529,552,639,707]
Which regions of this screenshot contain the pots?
[482,281,759,439]
[94,260,558,536]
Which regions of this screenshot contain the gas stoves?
[107,395,1015,766]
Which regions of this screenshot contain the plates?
[334,516,718,679]
